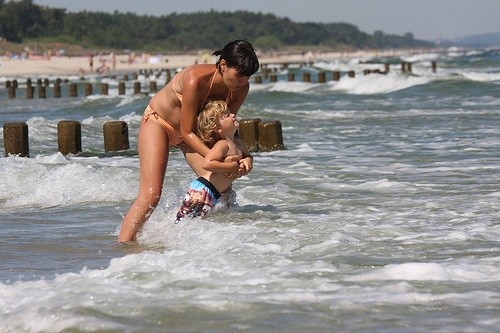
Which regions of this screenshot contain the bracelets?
[246,155,253,159]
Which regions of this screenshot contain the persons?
[117,39,259,244]
[6,47,209,73]
[177,101,254,222]
